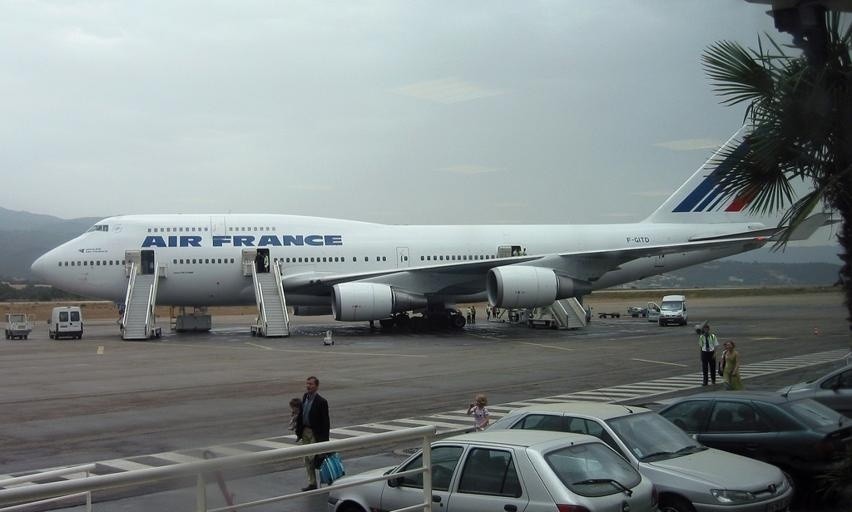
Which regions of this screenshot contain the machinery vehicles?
[626,306,644,318]
[6,311,33,339]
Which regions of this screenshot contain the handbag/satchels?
[314,452,345,485]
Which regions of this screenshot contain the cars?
[324,428,660,511]
[653,389,851,491]
[778,364,852,416]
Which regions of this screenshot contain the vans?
[646,294,688,327]
[47,304,84,339]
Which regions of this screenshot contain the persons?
[287,399,304,444]
[464,303,501,324]
[254,251,263,273]
[294,376,330,491]
[262,254,270,273]
[465,395,489,432]
[513,247,528,256]
[718,339,747,391]
[696,326,720,386]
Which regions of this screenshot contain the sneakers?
[302,484,317,490]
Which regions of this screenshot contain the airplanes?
[29,125,845,337]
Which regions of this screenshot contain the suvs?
[482,401,794,511]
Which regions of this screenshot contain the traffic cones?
[813,325,819,337]
